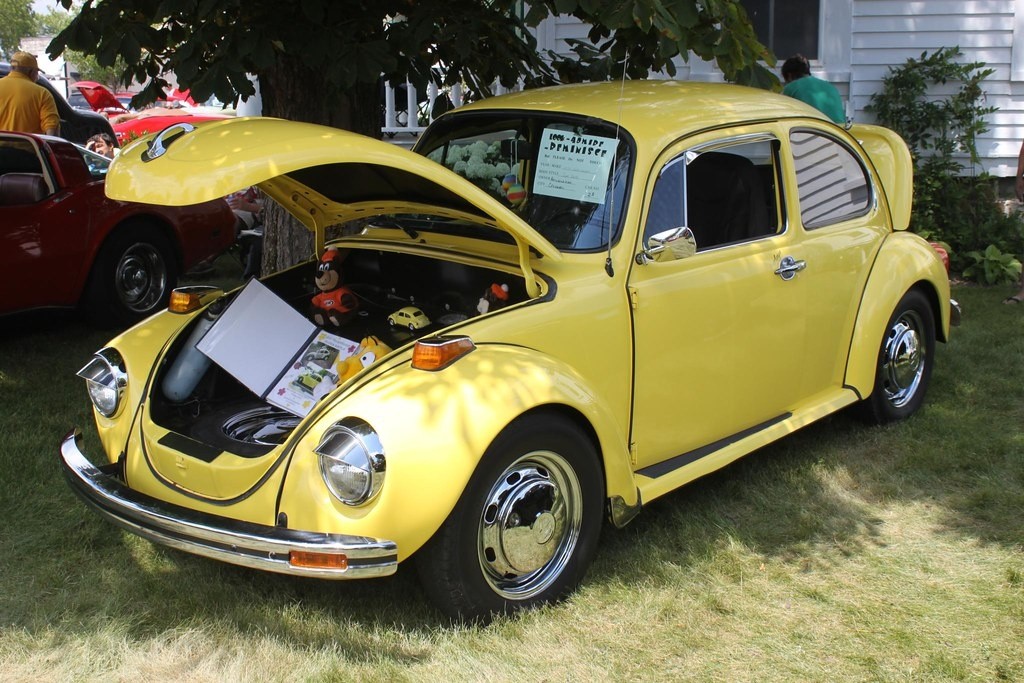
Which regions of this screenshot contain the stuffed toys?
[502,173,527,207]
[477,283,510,315]
[310,245,360,330]
[313,335,393,405]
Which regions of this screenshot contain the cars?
[60,79,962,615]
[1,128,238,321]
[0,64,238,143]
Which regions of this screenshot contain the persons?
[0,52,61,138]
[188,185,267,272]
[781,53,847,124]
[84,133,121,171]
[162,82,178,108]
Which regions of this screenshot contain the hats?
[11,52,45,75]
